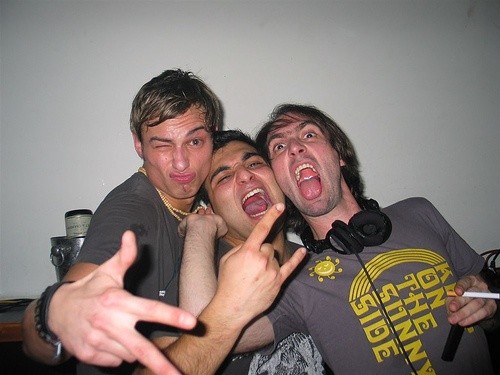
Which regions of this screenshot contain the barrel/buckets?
[51,237,85,282]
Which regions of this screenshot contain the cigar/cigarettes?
[198,197,214,215]
[447,291,500,299]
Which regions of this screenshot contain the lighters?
[441,326,464,362]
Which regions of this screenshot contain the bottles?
[65,209,93,238]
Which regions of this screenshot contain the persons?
[22,70,217,375]
[129,130,325,375]
[178,103,500,375]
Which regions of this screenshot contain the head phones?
[300,198,393,255]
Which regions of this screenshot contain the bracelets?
[138,167,202,221]
[34,276,75,364]
